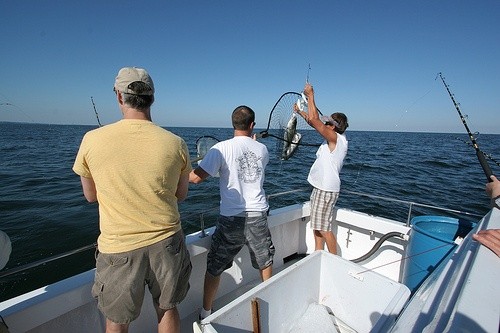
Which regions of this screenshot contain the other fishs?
[282,116,301,160]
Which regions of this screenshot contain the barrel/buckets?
[402,216,477,297]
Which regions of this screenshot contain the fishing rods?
[433,70,496,184]
[89,95,102,127]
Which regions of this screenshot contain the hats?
[321,115,341,131]
[113,67,156,95]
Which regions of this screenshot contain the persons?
[292,82,348,255]
[72,67,193,333]
[486,175,500,209]
[472,228,500,258]
[189,105,275,324]
[0,230,12,333]
